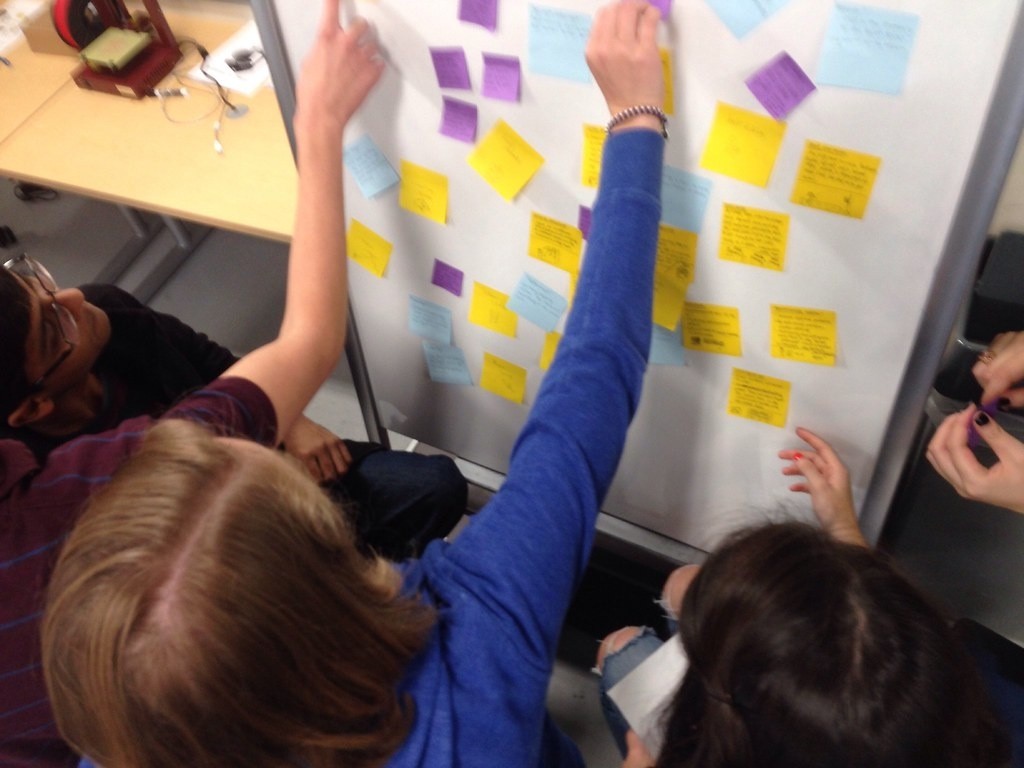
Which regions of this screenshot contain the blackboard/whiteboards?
[248,0,1024,569]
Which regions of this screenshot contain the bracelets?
[606,105,667,137]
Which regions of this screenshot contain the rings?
[981,350,993,363]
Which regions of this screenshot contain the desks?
[0,0,298,304]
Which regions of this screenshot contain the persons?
[924,330,1024,516]
[0,0,467,768]
[593,428,1011,767]
[43,0,662,768]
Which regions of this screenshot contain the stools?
[899,233,1023,509]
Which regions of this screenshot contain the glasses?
[4,253,81,394]
